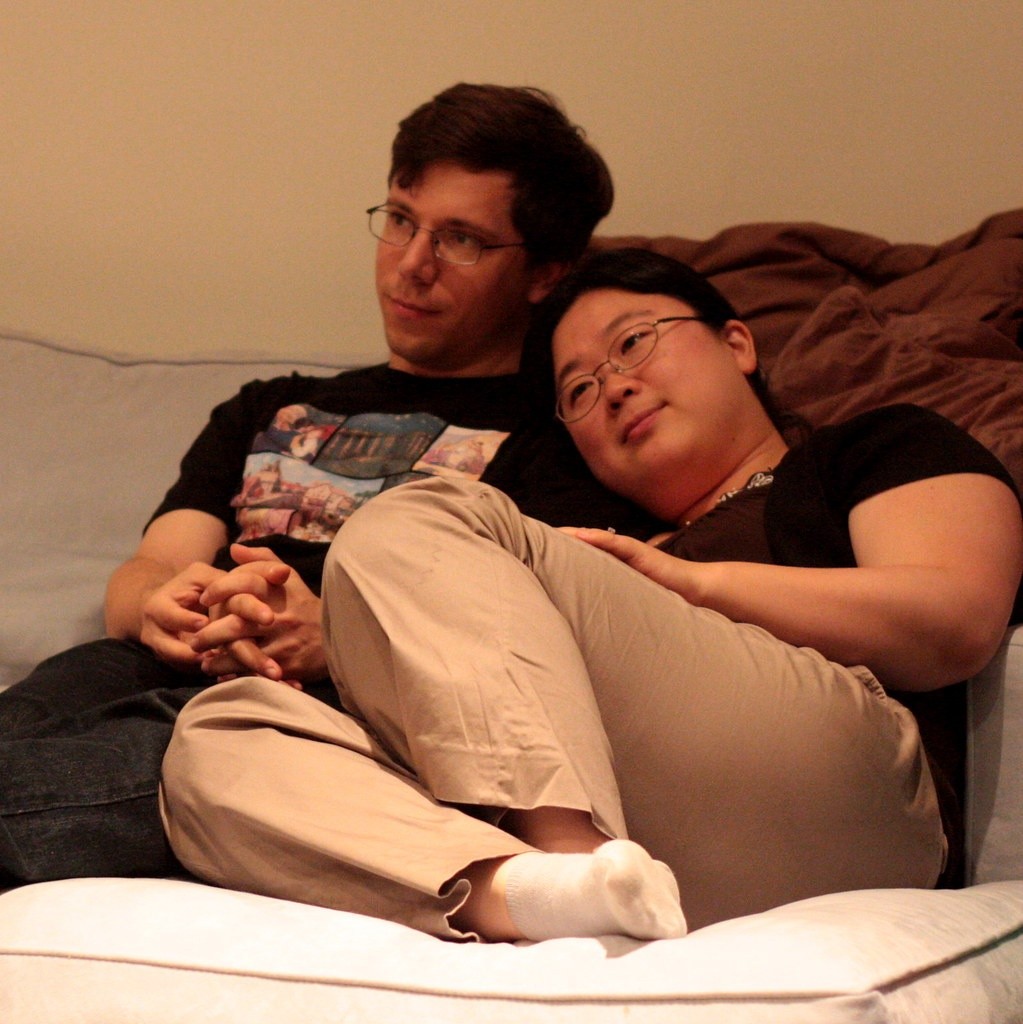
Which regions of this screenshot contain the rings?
[608,527,615,533]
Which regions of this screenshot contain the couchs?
[0,329,1023,1024]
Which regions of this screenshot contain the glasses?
[367,202,529,265]
[554,316,711,423]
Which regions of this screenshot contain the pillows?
[769,288,1023,495]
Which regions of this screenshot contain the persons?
[160,246,1023,943]
[0,83,616,894]
